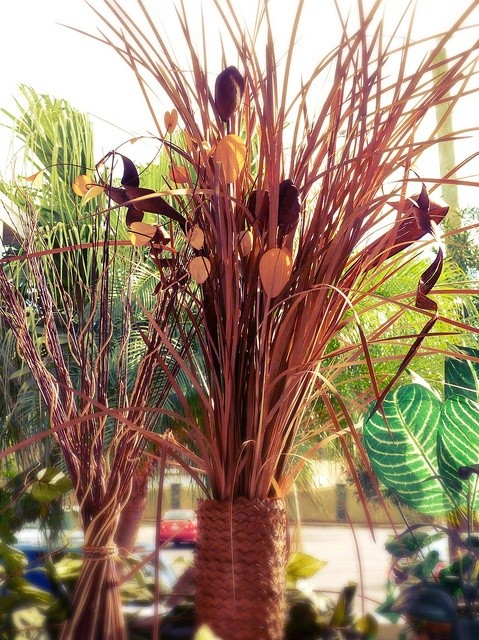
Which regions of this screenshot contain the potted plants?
[361,338,479,640]
